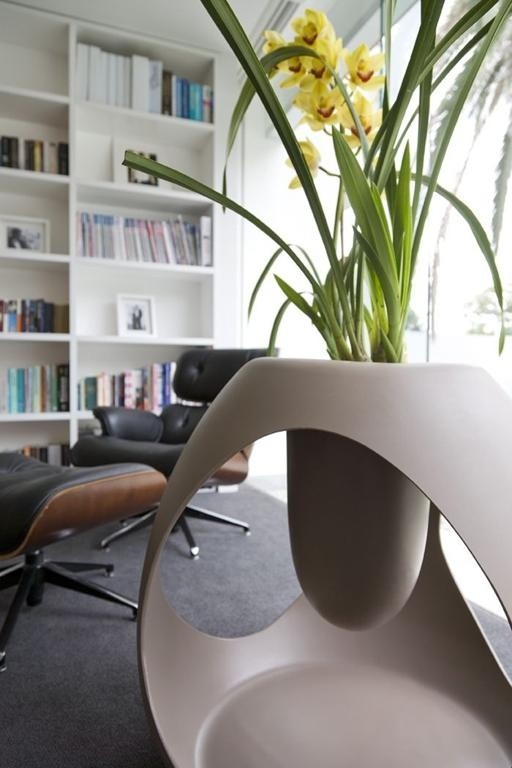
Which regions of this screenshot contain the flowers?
[127,0,512,373]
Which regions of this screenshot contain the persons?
[8,228,27,249]
[133,305,142,330]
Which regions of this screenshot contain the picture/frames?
[116,293,158,340]
[111,136,168,192]
[0,214,53,254]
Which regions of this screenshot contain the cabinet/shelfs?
[0,0,218,461]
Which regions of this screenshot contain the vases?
[136,357,512,768]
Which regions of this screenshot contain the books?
[2,297,70,334]
[76,42,211,122]
[0,134,69,176]
[7,362,203,413]
[76,207,211,266]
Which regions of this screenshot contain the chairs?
[72,343,283,558]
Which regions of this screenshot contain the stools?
[2,448,174,670]
